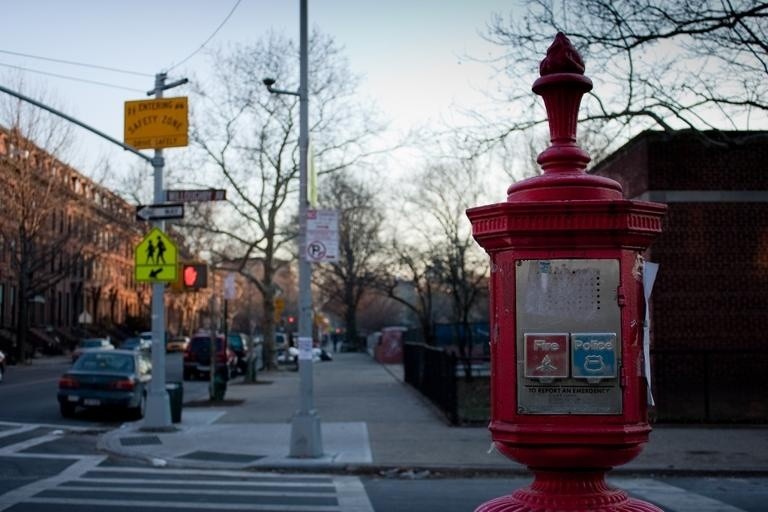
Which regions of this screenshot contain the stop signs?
[182,263,207,290]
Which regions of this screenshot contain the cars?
[56,347,152,420]
[70,337,117,363]
[119,327,297,380]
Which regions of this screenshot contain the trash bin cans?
[165,381,183,423]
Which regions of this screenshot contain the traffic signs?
[135,203,183,221]
